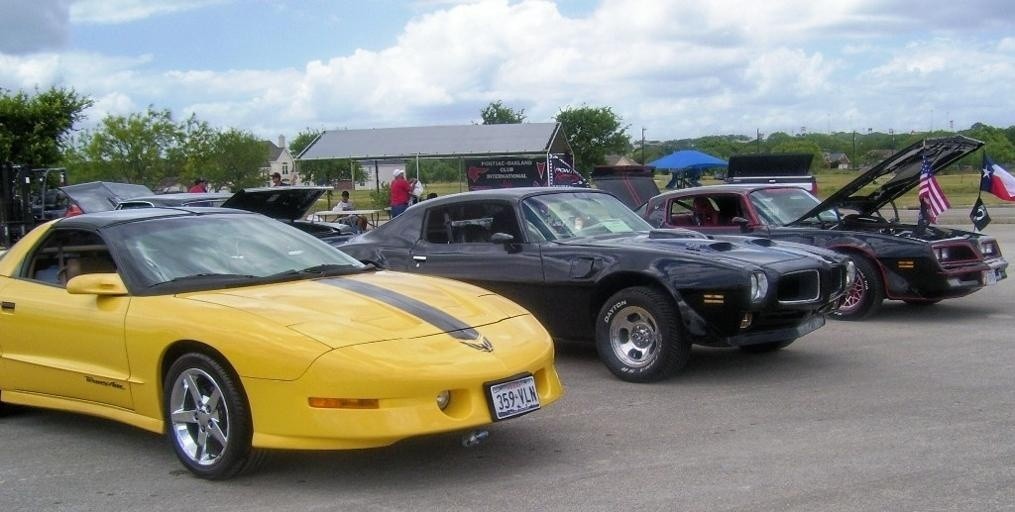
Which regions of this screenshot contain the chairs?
[59,257,113,287]
[692,196,719,226]
[453,204,546,243]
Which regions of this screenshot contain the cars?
[60,180,356,246]
[28,186,83,223]
[725,152,817,197]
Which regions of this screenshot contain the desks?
[315,209,381,229]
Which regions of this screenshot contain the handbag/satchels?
[407,178,424,196]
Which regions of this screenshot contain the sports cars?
[330,186,857,383]
[631,133,1009,320]
[4,206,554,481]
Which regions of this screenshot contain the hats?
[269,172,280,177]
[393,168,405,177]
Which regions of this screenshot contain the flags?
[979,148,1014,202]
[919,148,952,227]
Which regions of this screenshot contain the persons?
[269,172,289,187]
[335,191,368,235]
[189,179,209,207]
[389,169,417,219]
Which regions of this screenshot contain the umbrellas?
[644,149,729,189]
